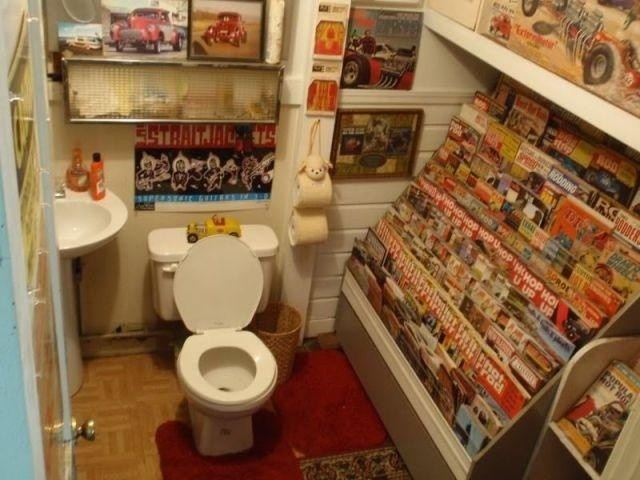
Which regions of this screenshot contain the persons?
[354,29,377,59]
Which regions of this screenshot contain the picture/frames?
[327,106,425,182]
[185,1,268,62]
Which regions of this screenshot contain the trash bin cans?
[249,301,302,384]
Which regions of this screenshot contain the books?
[346,71,640,478]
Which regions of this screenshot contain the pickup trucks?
[110,7,185,53]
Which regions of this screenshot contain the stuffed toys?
[297,156,335,181]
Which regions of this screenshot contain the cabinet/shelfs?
[526,336,640,480]
[330,5,639,479]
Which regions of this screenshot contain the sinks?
[55,185,129,258]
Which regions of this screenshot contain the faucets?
[53,191,65,198]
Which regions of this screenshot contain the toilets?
[146,225,280,456]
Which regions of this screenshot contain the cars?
[66,36,101,50]
[341,43,416,89]
[205,9,247,47]
[488,12,512,39]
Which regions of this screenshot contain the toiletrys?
[90,152,106,200]
